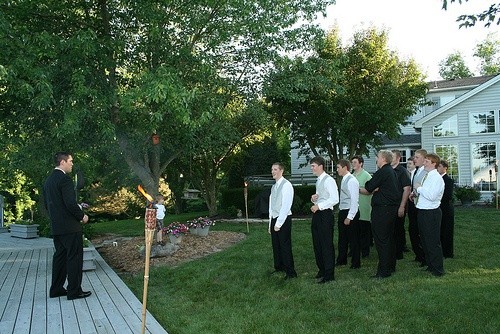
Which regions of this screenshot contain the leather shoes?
[78,290,92,298]
[50,290,68,298]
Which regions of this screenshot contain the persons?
[347,156,373,258]
[390,150,411,259]
[310,157,339,283]
[268,163,298,280]
[335,160,361,269]
[407,149,454,277]
[359,149,401,278]
[42,152,92,300]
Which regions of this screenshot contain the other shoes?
[310,273,321,279]
[369,274,388,279]
[283,276,295,280]
[314,278,329,284]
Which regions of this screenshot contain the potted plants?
[82,235,96,270]
[9,219,40,238]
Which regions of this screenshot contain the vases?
[189,226,210,236]
[169,234,182,244]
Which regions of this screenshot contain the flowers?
[187,215,212,229]
[163,221,189,238]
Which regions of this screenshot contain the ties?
[411,166,419,193]
[415,173,428,206]
